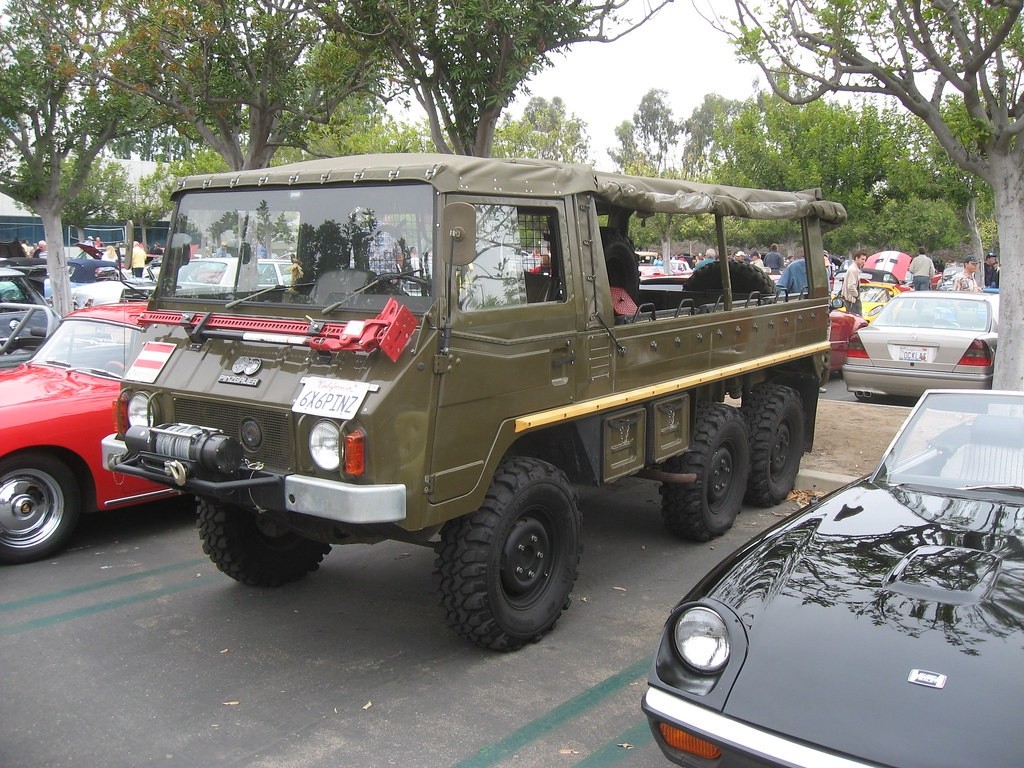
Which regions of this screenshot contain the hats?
[986,253,997,257]
[964,255,978,263]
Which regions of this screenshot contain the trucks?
[100,152,849,653]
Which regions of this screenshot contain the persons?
[131,241,147,278]
[256,241,267,259]
[974,252,999,289]
[216,242,227,258]
[953,255,983,293]
[673,244,848,273]
[909,246,935,291]
[101,246,118,262]
[145,242,162,264]
[21,239,47,258]
[840,251,866,316]
[776,259,831,392]
[95,236,103,247]
[86,236,94,247]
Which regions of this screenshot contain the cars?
[0,303,196,566]
[842,290,1001,400]
[633,250,1002,373]
[640,387,1024,767]
[0,240,293,369]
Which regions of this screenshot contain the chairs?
[479,273,555,307]
[956,311,976,327]
[939,416,1023,485]
[916,309,933,324]
[898,308,918,327]
[308,269,378,302]
